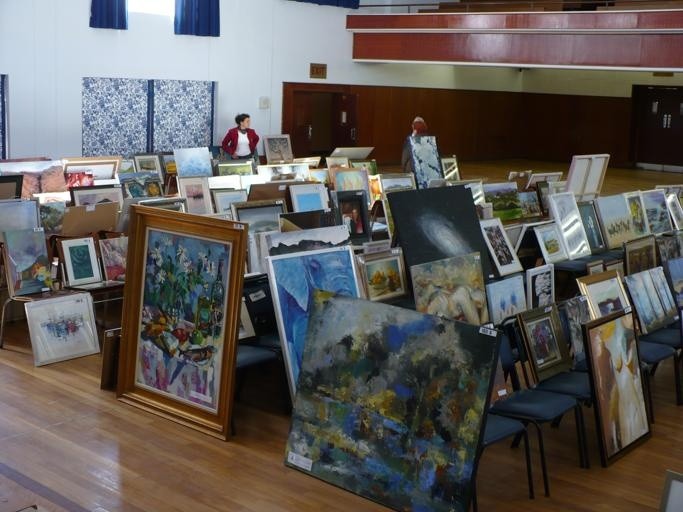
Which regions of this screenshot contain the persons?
[221,112,259,162]
[399,115,429,174]
